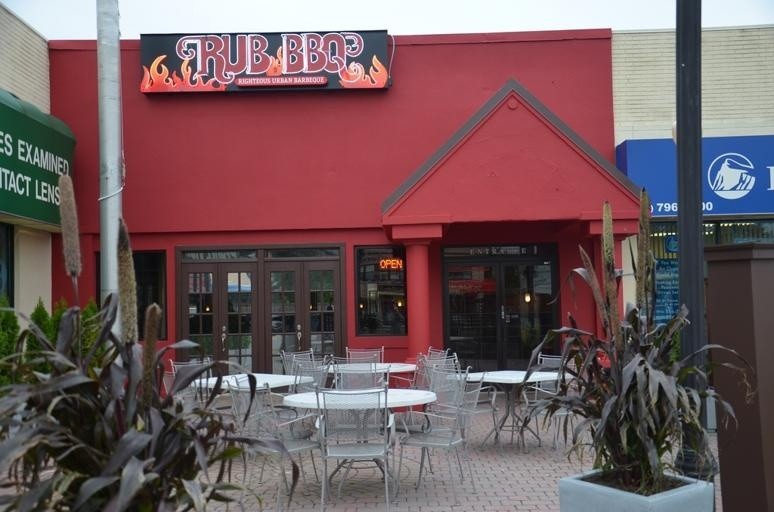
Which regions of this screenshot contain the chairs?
[401,364,486,492]
[310,383,399,511]
[161,344,602,451]
[225,372,321,512]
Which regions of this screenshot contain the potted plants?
[498,191,749,512]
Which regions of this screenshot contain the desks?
[280,387,438,499]
